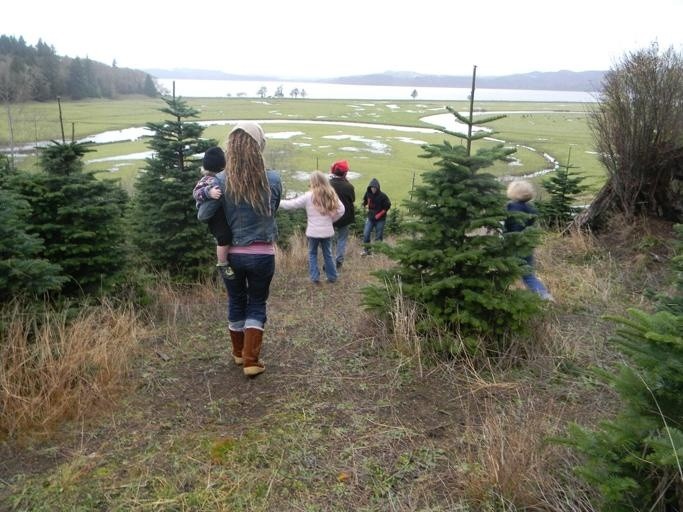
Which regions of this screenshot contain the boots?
[229,326,265,376]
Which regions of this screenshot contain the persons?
[190,146,234,279]
[322,158,356,272]
[500,177,555,307]
[195,122,281,378]
[273,170,345,285]
[360,178,390,257]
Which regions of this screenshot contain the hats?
[203,146,225,172]
[507,181,535,202]
[332,159,350,172]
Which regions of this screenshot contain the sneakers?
[358,249,371,258]
[216,264,236,280]
[335,258,344,267]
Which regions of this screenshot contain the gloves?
[374,213,382,220]
